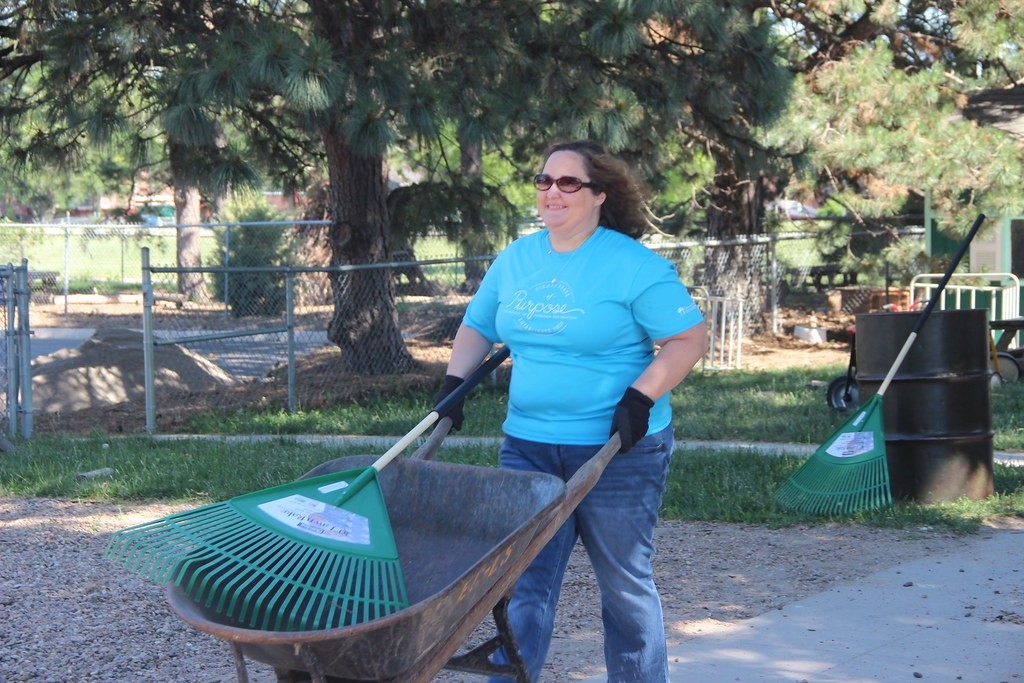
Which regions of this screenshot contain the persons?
[432,140,708,683]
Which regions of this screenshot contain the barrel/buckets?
[852,309,994,505]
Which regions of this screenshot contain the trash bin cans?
[854,309,995,502]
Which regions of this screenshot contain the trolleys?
[165,416,623,683]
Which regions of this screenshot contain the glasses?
[533,174,591,193]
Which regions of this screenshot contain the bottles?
[809,310,817,329]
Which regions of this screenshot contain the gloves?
[433,375,465,436]
[609,385,655,454]
